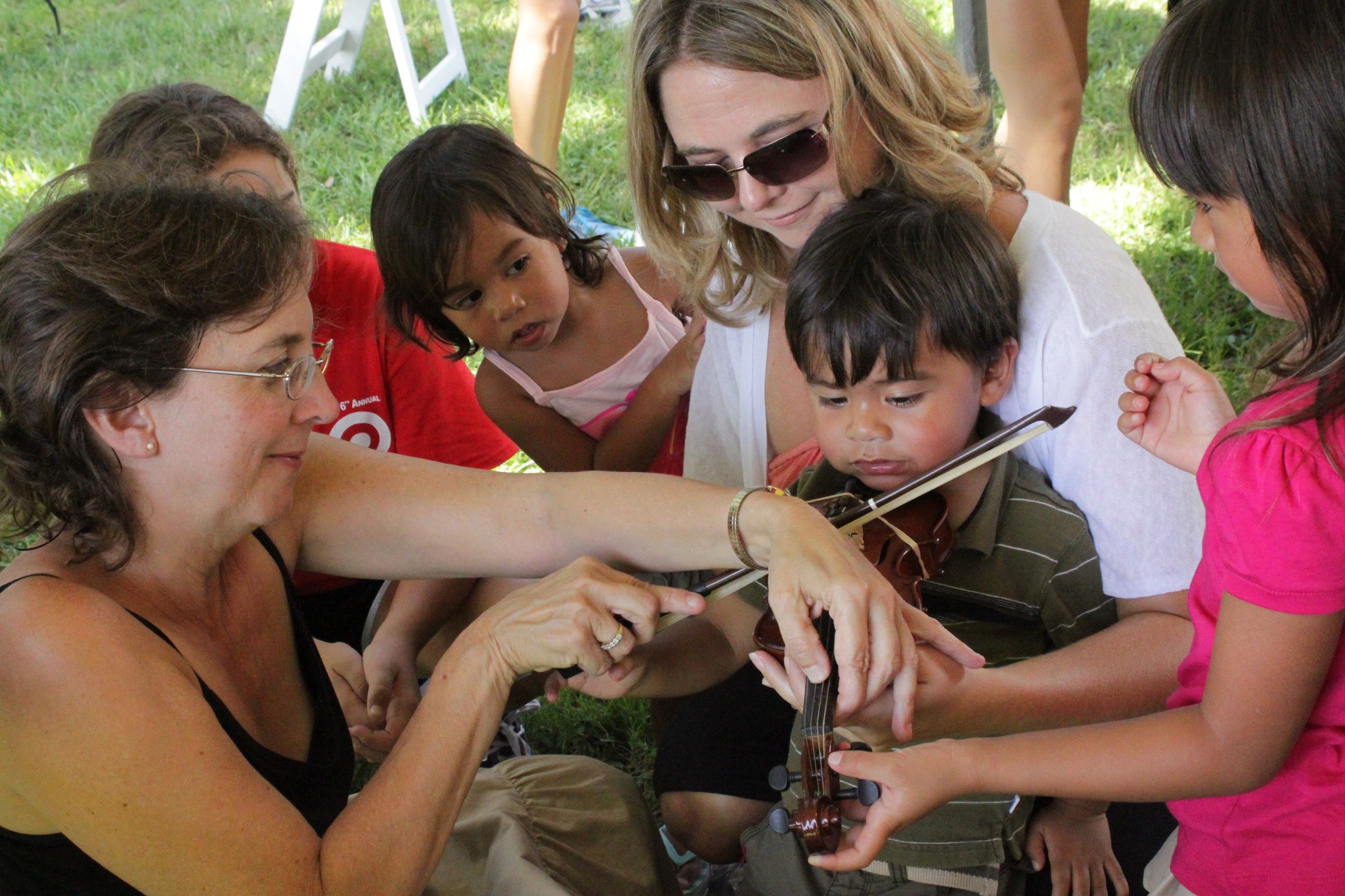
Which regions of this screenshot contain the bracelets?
[728,485,789,570]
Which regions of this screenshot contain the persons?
[366,1,1344,896]
[88,84,544,771]
[0,163,986,896]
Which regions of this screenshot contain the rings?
[601,621,623,650]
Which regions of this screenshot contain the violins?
[753,477,951,857]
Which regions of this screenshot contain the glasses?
[660,102,837,201]
[142,339,333,401]
